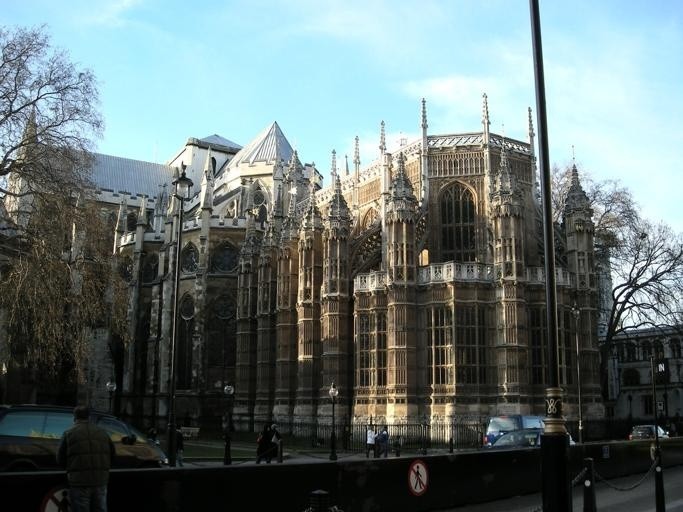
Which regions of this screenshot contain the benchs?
[181,426,200,440]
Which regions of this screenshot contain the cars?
[0,405,170,469]
[629,425,669,439]
[482,415,576,450]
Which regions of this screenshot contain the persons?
[165,423,185,468]
[364,425,376,456]
[55,403,115,511]
[379,424,389,432]
[251,423,274,463]
[376,429,389,458]
[262,422,282,460]
[338,426,354,453]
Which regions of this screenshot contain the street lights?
[106,376,117,414]
[169,160,195,423]
[223,382,234,464]
[571,301,584,443]
[328,379,339,460]
